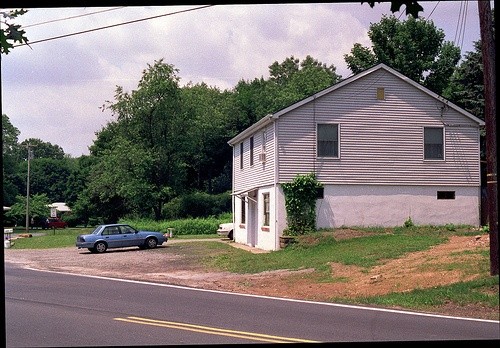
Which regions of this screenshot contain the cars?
[76,223,168,253]
[217,222,233,240]
[46,217,68,229]
